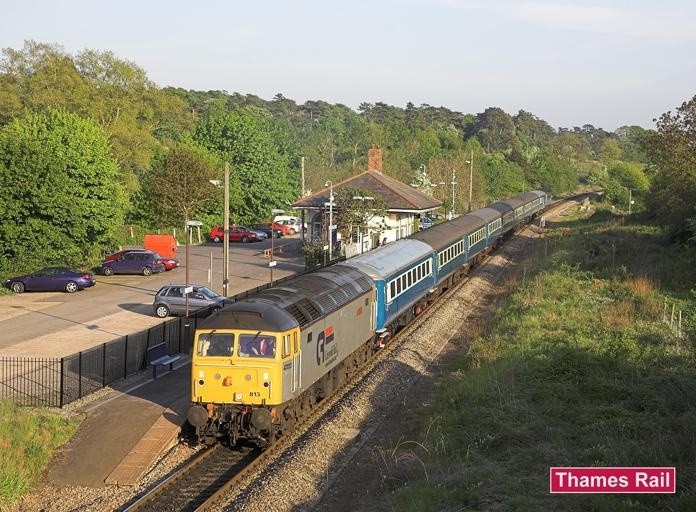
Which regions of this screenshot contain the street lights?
[353,194,377,262]
[420,163,427,190]
[267,208,285,289]
[185,218,205,318]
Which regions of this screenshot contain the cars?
[104,249,180,272]
[4,266,96,294]
[207,215,306,243]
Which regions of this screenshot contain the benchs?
[145,341,179,377]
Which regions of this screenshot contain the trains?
[184,189,548,455]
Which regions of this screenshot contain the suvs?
[101,252,162,276]
[152,283,236,319]
